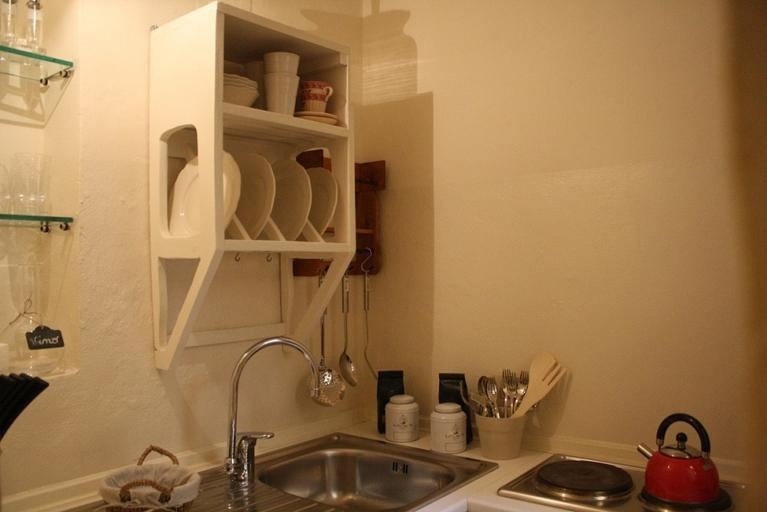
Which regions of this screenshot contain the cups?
[263,72,299,117]
[263,53,300,74]
[475,410,527,460]
[298,80,333,112]
[0,154,52,214]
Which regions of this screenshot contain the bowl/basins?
[224,72,260,107]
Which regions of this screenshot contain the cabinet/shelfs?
[0,37,75,226]
[149,2,357,370]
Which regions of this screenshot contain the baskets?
[100,446,199,511]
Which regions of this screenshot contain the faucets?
[225,338,320,481]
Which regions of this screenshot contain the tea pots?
[633,412,720,505]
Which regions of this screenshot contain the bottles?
[1,0,18,46]
[383,393,421,443]
[26,1,44,53]
[429,403,466,454]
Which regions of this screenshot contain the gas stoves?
[493,450,753,511]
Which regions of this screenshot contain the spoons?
[477,375,500,419]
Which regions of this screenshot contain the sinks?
[254,431,499,512]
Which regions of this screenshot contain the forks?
[500,368,528,418]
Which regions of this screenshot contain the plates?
[308,165,339,237]
[226,153,278,239]
[166,150,241,238]
[272,158,313,240]
[294,111,340,126]
